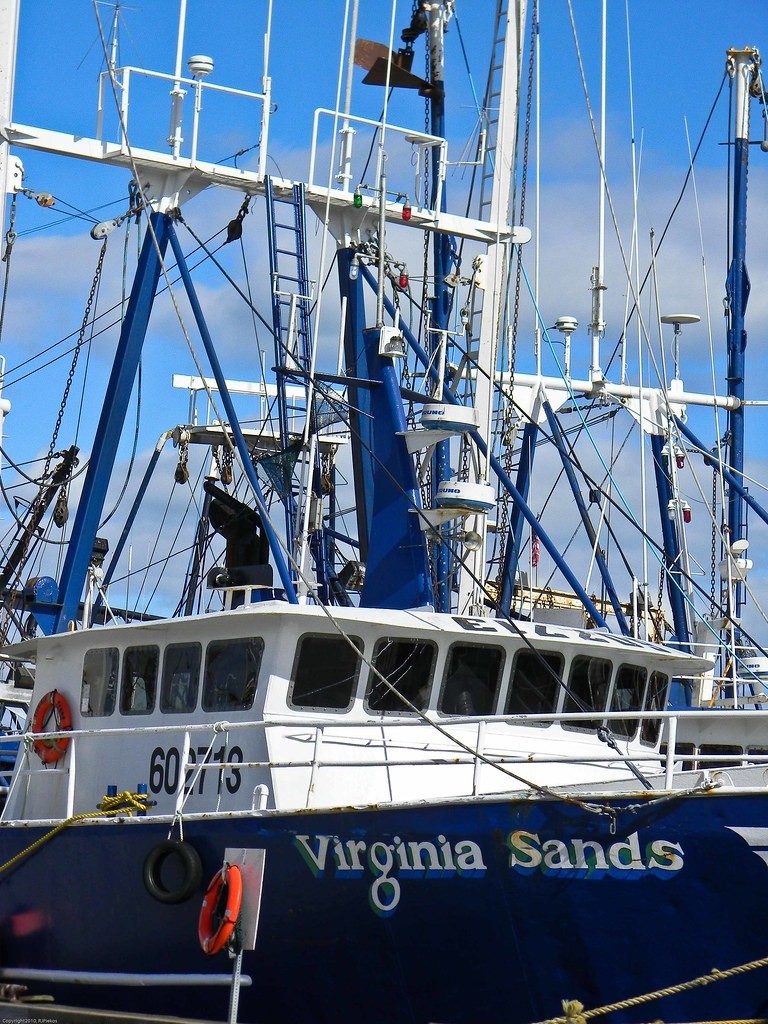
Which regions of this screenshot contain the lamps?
[667,502,676,520]
[682,503,692,523]
[349,258,360,280]
[353,187,362,208]
[660,445,670,466]
[403,198,411,220]
[676,450,685,468]
[401,267,409,287]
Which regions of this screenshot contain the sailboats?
[0,0,766,1023]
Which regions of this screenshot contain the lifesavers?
[140,837,201,904]
[194,863,245,956]
[32,690,71,763]
